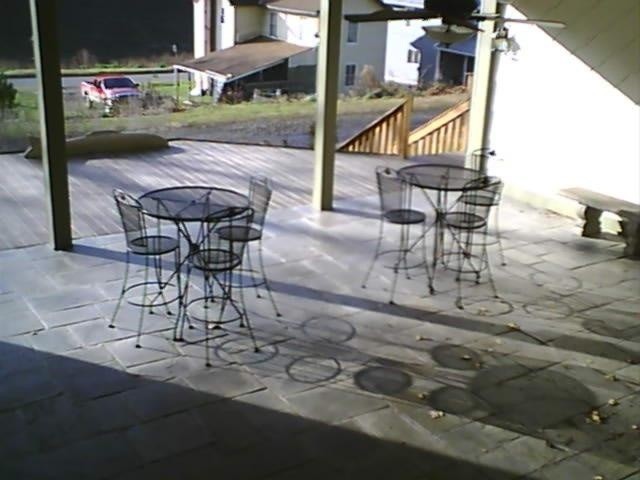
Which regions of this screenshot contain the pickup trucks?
[81,75,145,108]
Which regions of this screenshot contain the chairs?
[360,160,506,310]
[107,177,280,371]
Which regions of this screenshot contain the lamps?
[487,22,510,53]
[421,9,476,48]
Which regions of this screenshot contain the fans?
[343,0,570,33]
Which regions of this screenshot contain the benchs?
[557,182,638,252]
